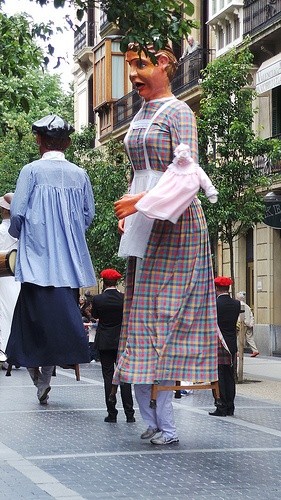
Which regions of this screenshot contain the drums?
[0,248,17,277]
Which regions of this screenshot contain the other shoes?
[141,428,179,444]
[104,413,117,423]
[209,407,234,417]
[38,385,52,405]
[125,418,136,422]
[250,352,259,357]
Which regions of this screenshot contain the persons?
[234,290,259,359]
[27,364,55,405]
[0,193,21,362]
[208,276,240,417]
[112,36,219,383]
[89,269,135,423]
[78,291,95,323]
[3,115,87,368]
[132,382,180,445]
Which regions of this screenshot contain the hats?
[99,269,123,281]
[31,114,76,137]
[237,291,246,301]
[0,193,15,210]
[215,277,233,286]
